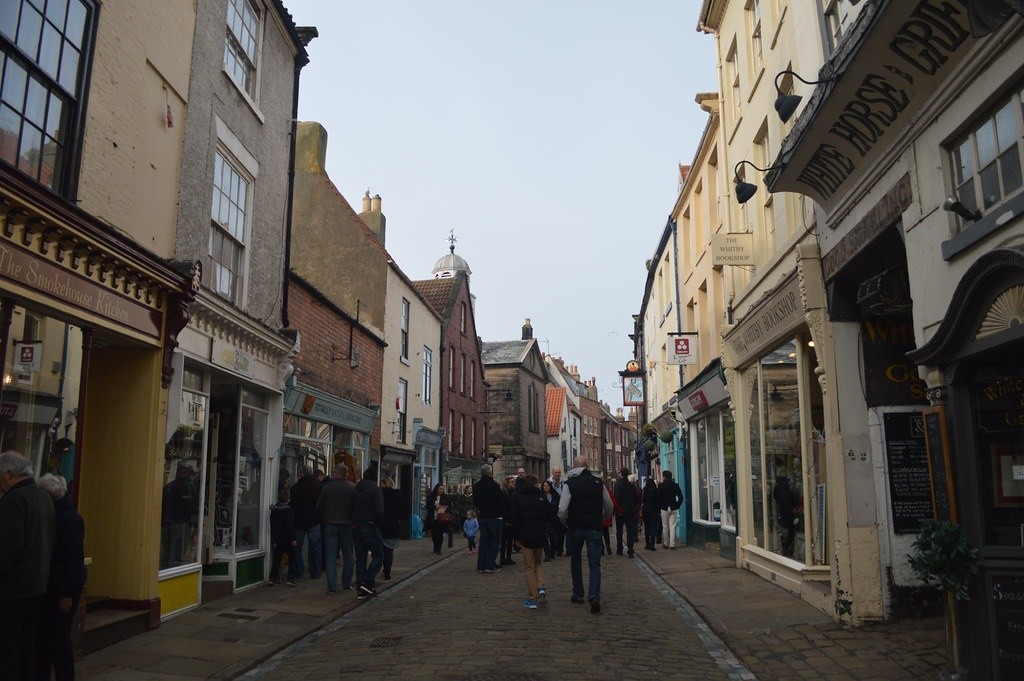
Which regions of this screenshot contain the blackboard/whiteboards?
[922,405,957,525]
[880,411,938,536]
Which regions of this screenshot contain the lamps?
[775,66,845,125]
[733,159,777,204]
[484,388,515,409]
[942,196,974,221]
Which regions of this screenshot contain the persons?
[162,463,195,568]
[456,485,473,523]
[0,450,57,681]
[348,468,384,600]
[289,464,324,578]
[499,467,526,566]
[316,463,356,594]
[463,510,479,555]
[558,455,612,613]
[473,464,505,573]
[422,483,451,555]
[642,479,658,551]
[614,468,637,558]
[773,477,799,556]
[512,473,552,608]
[601,485,624,556]
[269,489,297,587]
[541,466,570,557]
[657,470,684,549]
[38,473,86,681]
[378,477,404,582]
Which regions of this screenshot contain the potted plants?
[660,430,673,443]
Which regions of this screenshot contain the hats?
[620,467,629,477]
[334,461,349,475]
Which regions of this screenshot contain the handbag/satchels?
[437,512,452,525]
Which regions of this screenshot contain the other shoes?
[571,595,584,603]
[359,581,380,596]
[500,558,516,566]
[486,568,501,573]
[537,588,546,603]
[542,550,572,562]
[590,598,601,614]
[468,549,476,555]
[285,579,299,587]
[601,537,676,559]
[344,587,353,592]
[268,579,282,586]
[357,591,370,599]
[522,601,539,608]
[434,547,441,554]
